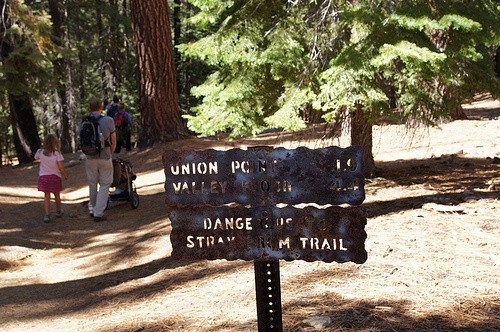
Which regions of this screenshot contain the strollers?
[107,158,139,209]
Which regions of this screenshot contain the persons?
[78,97,116,222]
[105,97,120,119]
[114,103,135,153]
[34,134,69,223]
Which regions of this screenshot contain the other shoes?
[43,215,50,224]
[94,214,109,223]
[55,210,62,218]
[90,213,94,218]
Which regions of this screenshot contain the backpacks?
[79,115,103,155]
[114,111,125,128]
[107,104,119,116]
